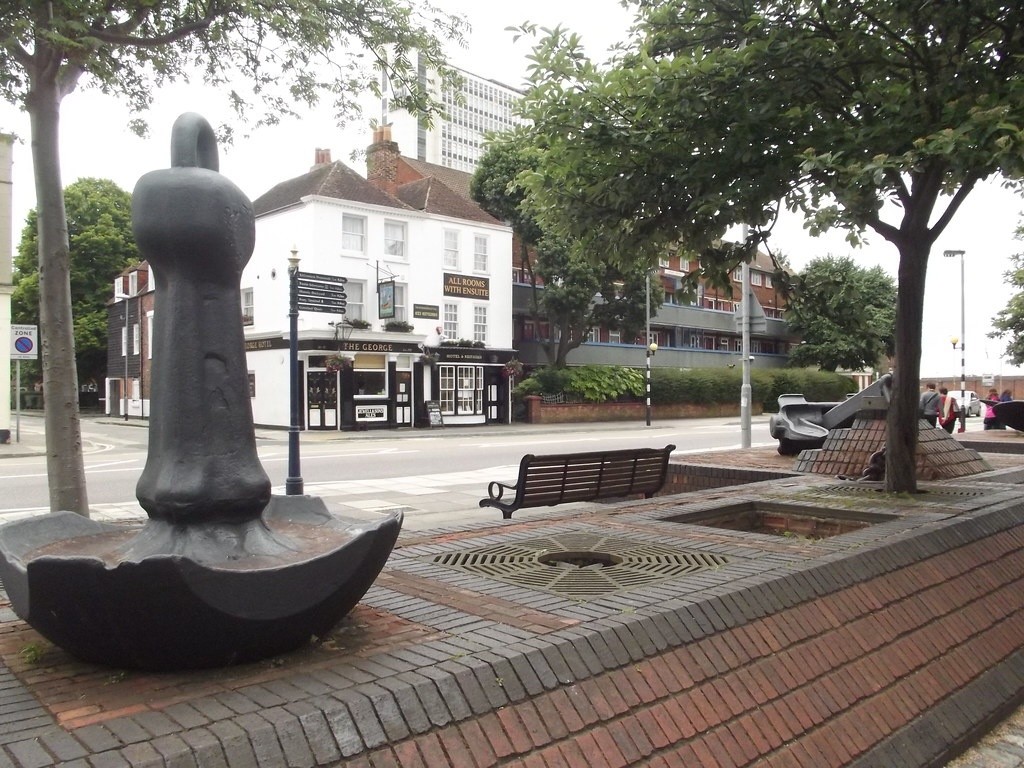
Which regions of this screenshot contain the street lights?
[944,249,966,431]
[115,293,131,420]
[644,266,659,424]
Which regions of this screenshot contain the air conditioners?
[720,338,728,345]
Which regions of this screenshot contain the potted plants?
[381,320,415,334]
[459,339,473,348]
[338,318,373,333]
[473,340,486,348]
[440,341,458,347]
[418,352,440,370]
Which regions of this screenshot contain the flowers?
[325,353,351,374]
[498,358,524,380]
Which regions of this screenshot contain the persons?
[936,387,961,434]
[984,387,1000,430]
[1000,389,1013,429]
[919,383,944,428]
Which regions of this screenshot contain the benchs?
[479,444,676,519]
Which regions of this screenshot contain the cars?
[947,391,981,417]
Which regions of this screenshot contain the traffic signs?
[298,272,348,314]
